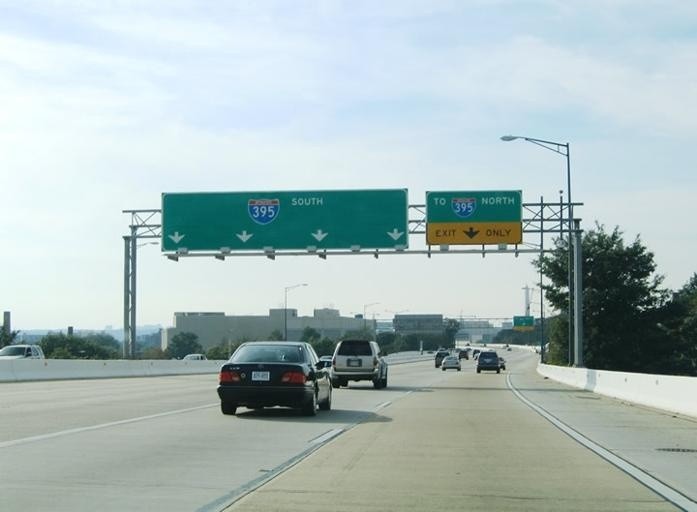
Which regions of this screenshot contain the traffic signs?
[511,315,536,331]
[156,189,409,251]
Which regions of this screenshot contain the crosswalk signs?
[426,190,522,245]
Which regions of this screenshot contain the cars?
[433,345,506,373]
[346,301,414,336]
[0,343,45,360]
[218,338,391,419]
[183,354,208,359]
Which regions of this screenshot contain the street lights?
[505,135,576,361]
[285,283,309,340]
